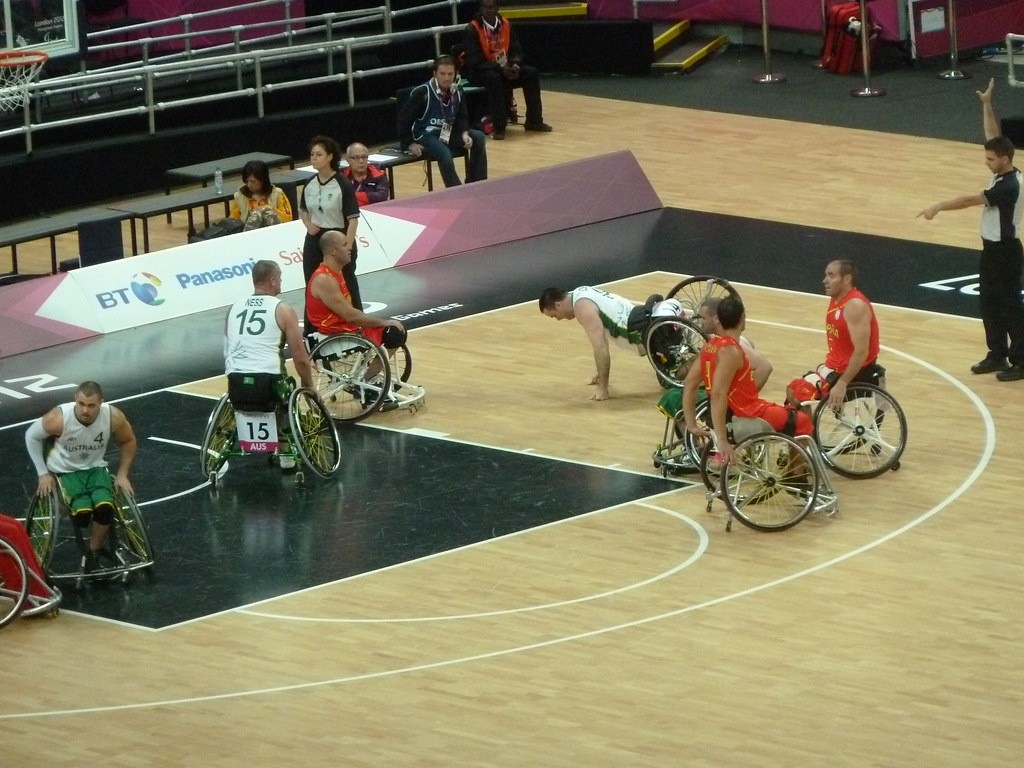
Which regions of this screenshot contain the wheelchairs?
[301,320,426,426]
[626,274,744,389]
[784,362,909,481]
[649,389,840,533]
[1,533,64,632]
[199,373,341,491]
[24,463,156,594]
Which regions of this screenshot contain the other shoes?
[996,364,1024,381]
[784,472,812,498]
[280,456,295,469]
[86,547,113,569]
[666,454,697,472]
[355,385,391,402]
[494,128,506,140]
[524,121,552,132]
[972,351,1010,374]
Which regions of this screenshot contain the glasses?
[349,154,368,160]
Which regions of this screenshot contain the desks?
[0,151,471,288]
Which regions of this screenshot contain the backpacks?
[821,1,864,75]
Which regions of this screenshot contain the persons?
[915,77,1024,382]
[305,230,407,404]
[397,55,487,188]
[784,260,880,423]
[655,295,814,496]
[538,284,686,400]
[300,137,364,329]
[462,0,554,140]
[222,259,325,469]
[228,160,292,223]
[25,381,137,558]
[343,142,388,206]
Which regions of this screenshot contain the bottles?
[214,166,223,194]
